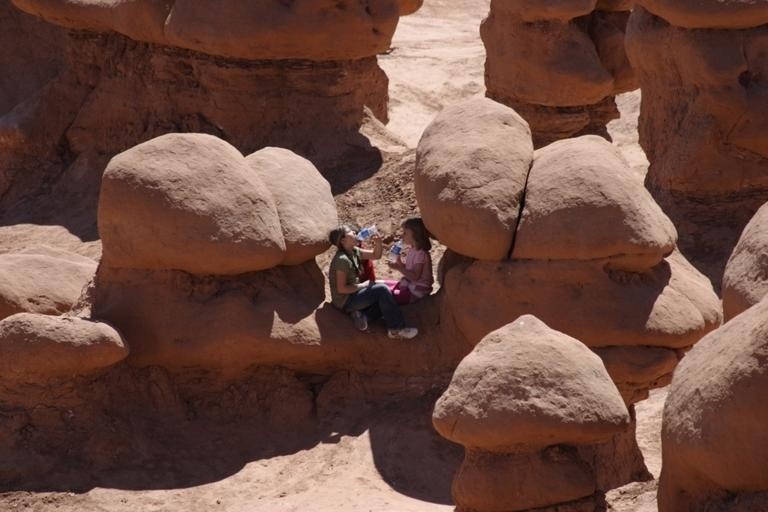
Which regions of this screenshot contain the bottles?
[355,225,379,242]
[389,239,404,263]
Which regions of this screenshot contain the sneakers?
[385,324,418,342]
[350,311,369,332]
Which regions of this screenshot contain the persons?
[373,217,434,303]
[328,225,419,340]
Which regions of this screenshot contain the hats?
[328,223,347,248]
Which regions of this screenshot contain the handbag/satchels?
[390,285,412,305]
[358,259,376,285]
